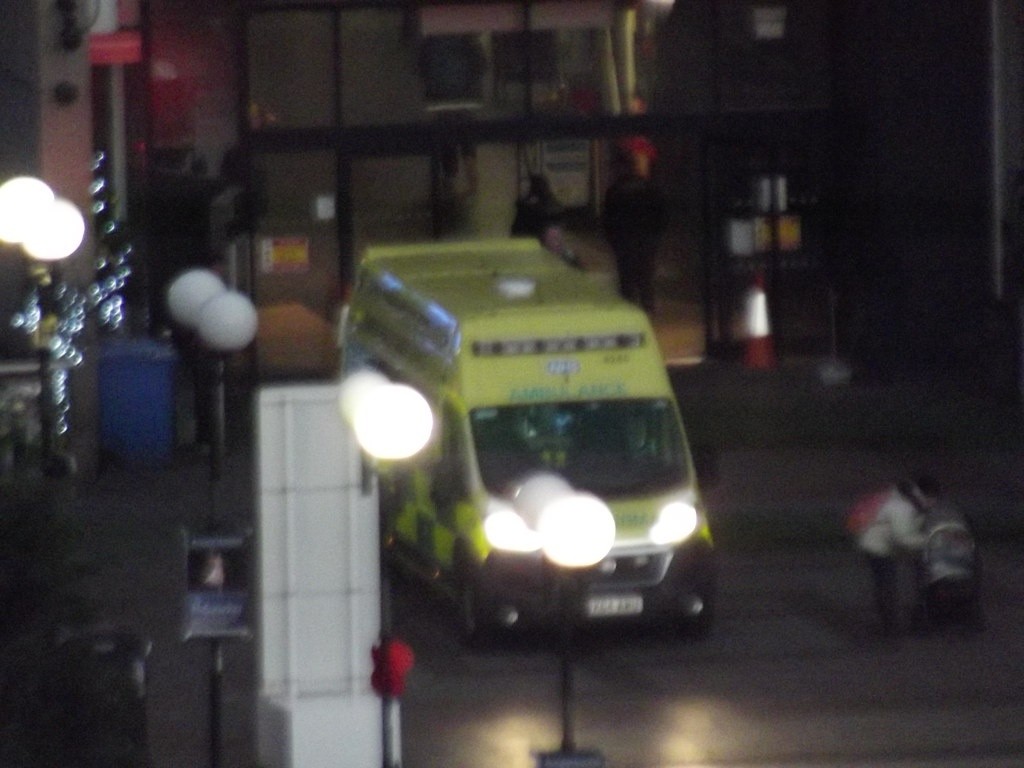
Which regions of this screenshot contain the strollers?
[910,504,991,632]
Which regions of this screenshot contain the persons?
[510,167,599,245]
[603,133,669,312]
[860,472,941,638]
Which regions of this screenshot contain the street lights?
[1,177,87,479]
[484,471,620,766]
[169,269,258,768]
[337,369,434,768]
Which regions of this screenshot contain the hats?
[902,477,943,508]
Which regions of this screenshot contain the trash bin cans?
[96,338,185,467]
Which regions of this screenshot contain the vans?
[334,238,720,642]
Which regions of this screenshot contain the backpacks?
[846,484,895,536]
